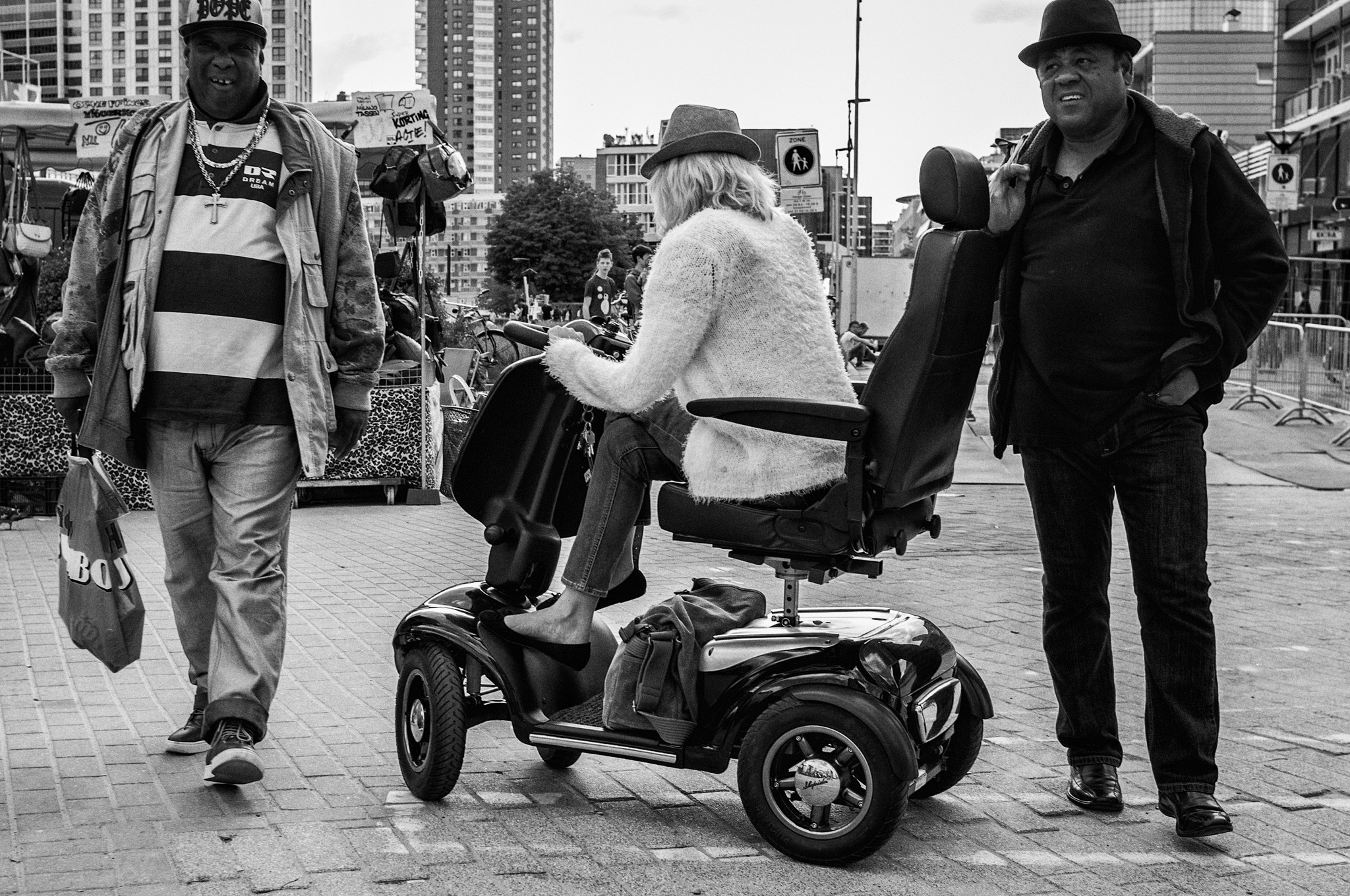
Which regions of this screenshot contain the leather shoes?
[1067,759,1125,814]
[1157,789,1235,838]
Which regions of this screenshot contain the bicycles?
[609,290,640,341]
[440,289,520,385]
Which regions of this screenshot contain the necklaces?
[185,99,273,224]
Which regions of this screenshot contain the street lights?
[512,257,539,325]
[847,98,872,256]
[1264,129,1305,238]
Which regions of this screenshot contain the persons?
[969,0,1293,834]
[582,249,617,327]
[46,0,387,785]
[488,309,497,324]
[841,321,879,369]
[503,296,584,323]
[624,243,650,327]
[480,101,871,672]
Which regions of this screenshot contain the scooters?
[392,145,1007,869]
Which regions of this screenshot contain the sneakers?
[202,719,266,785]
[165,693,212,754]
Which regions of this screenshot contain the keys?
[584,427,595,458]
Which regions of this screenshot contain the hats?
[1017,0,1141,68]
[640,104,759,181]
[176,1,269,45]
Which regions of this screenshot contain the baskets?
[439,403,480,499]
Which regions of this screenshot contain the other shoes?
[537,566,650,610]
[477,609,593,671]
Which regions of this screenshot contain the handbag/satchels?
[373,242,445,387]
[444,374,479,497]
[56,431,144,676]
[382,190,448,238]
[60,172,95,216]
[369,144,421,202]
[603,574,770,747]
[418,120,474,204]
[374,204,403,279]
[0,218,54,372]
